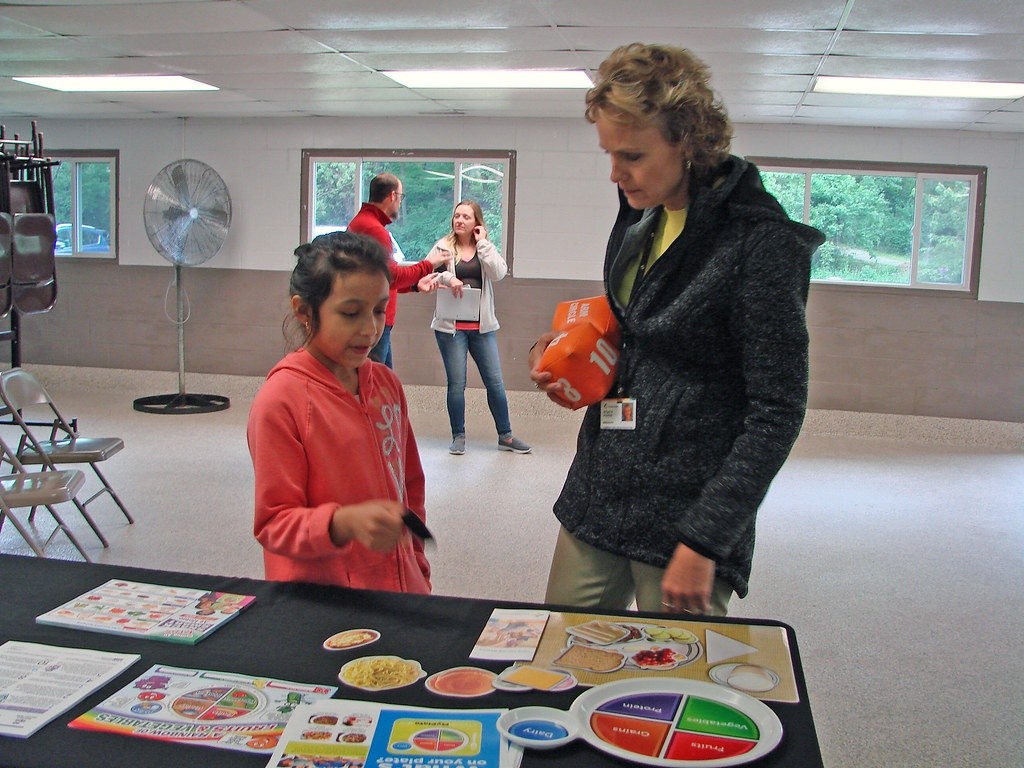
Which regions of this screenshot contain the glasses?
[388,193,405,201]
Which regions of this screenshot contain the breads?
[554,644,624,671]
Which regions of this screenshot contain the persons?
[528,42,826,616]
[246,231,433,594]
[424,200,532,455]
[345,172,453,369]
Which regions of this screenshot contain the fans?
[133,158,232,415]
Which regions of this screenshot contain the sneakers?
[498,435,531,454]
[449,437,466,454]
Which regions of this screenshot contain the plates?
[300,726,334,741]
[323,628,380,650]
[425,666,498,697]
[497,677,783,768]
[491,663,578,693]
[549,617,702,673]
[709,663,779,689]
[339,654,430,692]
[342,713,374,729]
[310,715,339,727]
[339,731,368,744]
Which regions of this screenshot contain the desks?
[0,554,826,768]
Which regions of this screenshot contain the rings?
[683,608,700,615]
[661,601,675,608]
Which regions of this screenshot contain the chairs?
[0,432,110,565]
[0,367,135,548]
[0,119,58,320]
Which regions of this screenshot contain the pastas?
[342,658,421,690]
